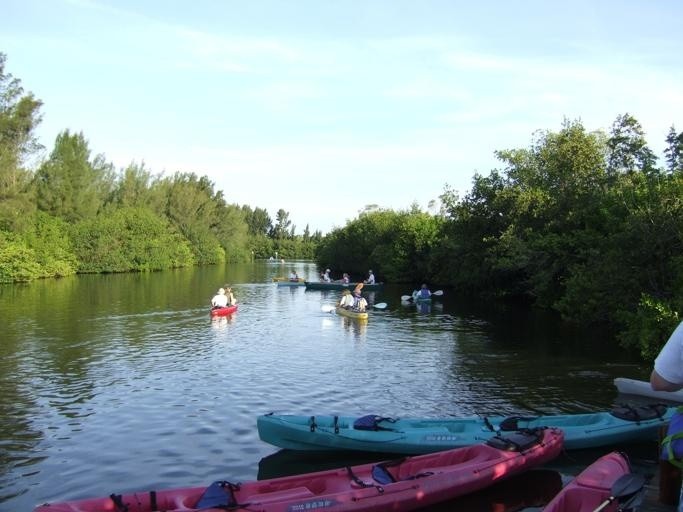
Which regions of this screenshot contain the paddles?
[592,474,650,512]
[366,301,388,309]
[400,290,443,301]
[322,305,341,312]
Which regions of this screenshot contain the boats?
[257,405,679,455]
[270,276,383,291]
[210,304,237,317]
[410,288,432,303]
[29,426,566,511]
[335,302,367,319]
[544,449,638,512]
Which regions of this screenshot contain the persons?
[651,320,683,512]
[290,271,298,282]
[415,284,432,303]
[211,287,236,309]
[320,255,375,313]
